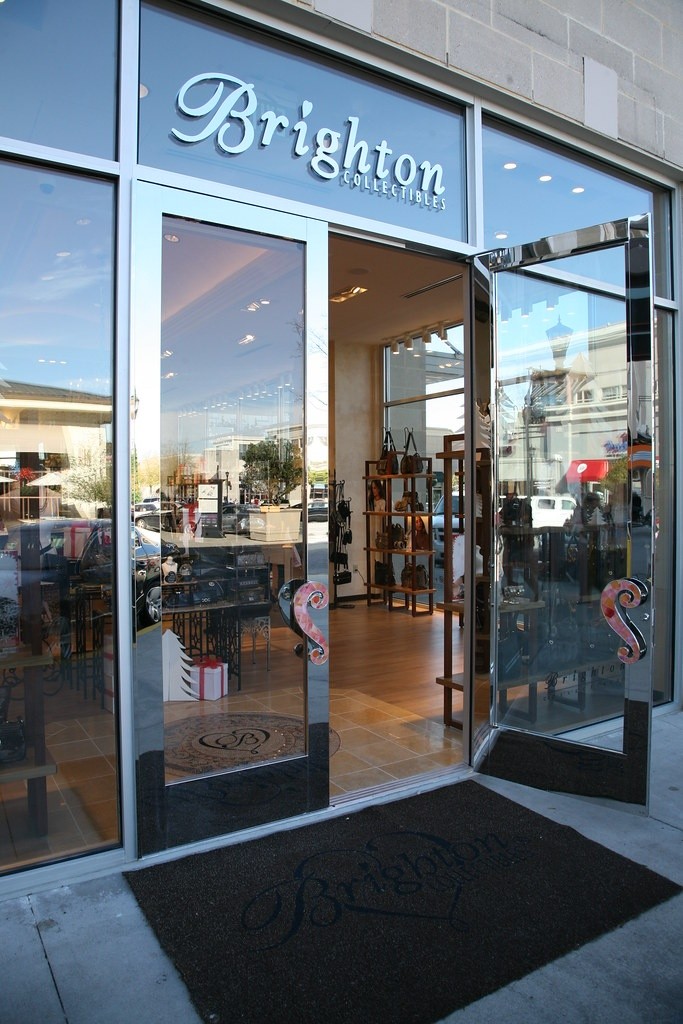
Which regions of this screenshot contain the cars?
[130,496,182,531]
[0,518,182,635]
[223,504,261,534]
[308,500,329,522]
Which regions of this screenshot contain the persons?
[569,493,609,603]
[497,488,540,600]
[0,465,63,622]
[407,515,430,550]
[367,480,386,512]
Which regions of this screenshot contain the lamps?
[545,314,574,370]
[392,321,448,355]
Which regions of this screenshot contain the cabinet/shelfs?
[362,435,633,730]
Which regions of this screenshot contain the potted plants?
[242,438,304,541]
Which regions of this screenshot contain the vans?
[433,489,465,562]
[498,494,577,529]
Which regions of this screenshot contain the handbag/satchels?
[79,552,113,582]
[330,483,352,585]
[387,524,405,549]
[376,431,398,474]
[375,561,395,586]
[41,553,68,582]
[165,517,273,618]
[475,492,642,683]
[401,432,423,474]
[401,563,428,590]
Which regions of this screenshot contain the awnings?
[565,460,609,484]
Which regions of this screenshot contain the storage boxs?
[190,662,228,700]
[102,635,115,713]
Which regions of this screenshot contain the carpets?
[164,711,339,778]
[122,781,683,1024]
